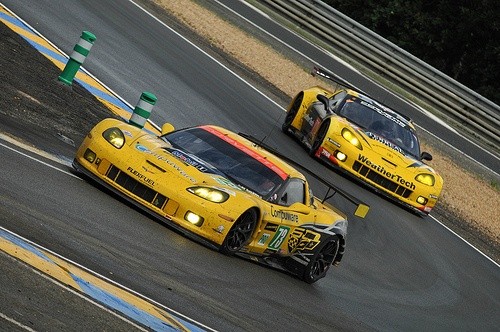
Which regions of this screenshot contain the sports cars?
[282,66,444,218]
[71,116,369,285]
